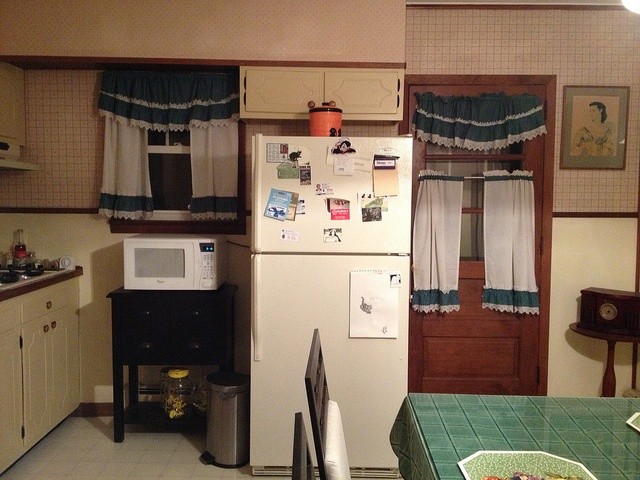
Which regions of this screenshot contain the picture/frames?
[558,84,630,170]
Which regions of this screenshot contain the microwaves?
[123,234,228,291]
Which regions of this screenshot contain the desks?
[389,393,639,480]
[569,322,640,397]
[106,284,240,443]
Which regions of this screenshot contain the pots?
[309,102,343,136]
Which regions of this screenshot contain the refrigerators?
[250,134,414,480]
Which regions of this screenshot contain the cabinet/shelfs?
[0,62,25,147]
[0,296,22,474]
[239,66,404,121]
[21,277,80,449]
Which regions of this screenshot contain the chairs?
[305,327,351,479]
[292,412,316,480]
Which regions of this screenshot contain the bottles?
[26,253,36,269]
[160,367,172,414]
[194,376,207,412]
[43,259,51,270]
[166,370,194,417]
[5,254,13,268]
[33,253,37,269]
[38,260,44,274]
[14,229,26,268]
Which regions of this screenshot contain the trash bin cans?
[200,371,250,469]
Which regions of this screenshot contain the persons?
[571,101,616,158]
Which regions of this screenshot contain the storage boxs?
[580,287,640,337]
